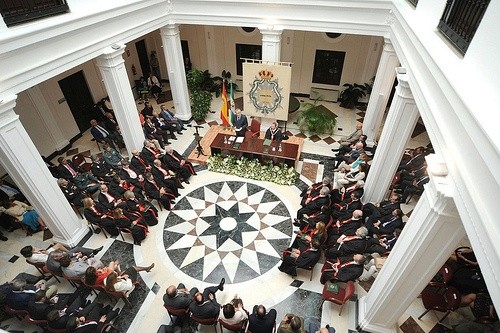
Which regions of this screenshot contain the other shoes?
[44,275,53,281]
[162,127,187,150]
[171,201,176,204]
[93,246,103,255]
[178,193,181,196]
[115,308,120,313]
[0,236,8,241]
[185,180,190,184]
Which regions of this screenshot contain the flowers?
[207,152,300,186]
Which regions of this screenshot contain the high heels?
[146,262,154,272]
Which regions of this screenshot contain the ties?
[238,117,240,123]
[66,164,77,176]
[149,123,153,127]
[271,128,278,141]
[97,126,109,137]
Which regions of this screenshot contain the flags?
[229,80,235,125]
[221,81,230,126]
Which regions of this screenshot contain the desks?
[210,133,299,169]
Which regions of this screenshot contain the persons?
[0,97,434,333]
[136,76,149,100]
[147,73,160,101]
[150,53,160,77]
[265,122,282,142]
[460,292,498,319]
[184,57,192,75]
[233,108,248,136]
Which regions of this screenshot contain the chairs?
[0,259,146,333]
[281,149,498,333]
[65,153,195,243]
[163,304,277,333]
[244,119,261,139]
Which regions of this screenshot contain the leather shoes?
[219,278,225,291]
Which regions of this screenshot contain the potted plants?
[338,82,369,108]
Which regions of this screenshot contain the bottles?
[278,144,283,152]
[223,136,227,144]
